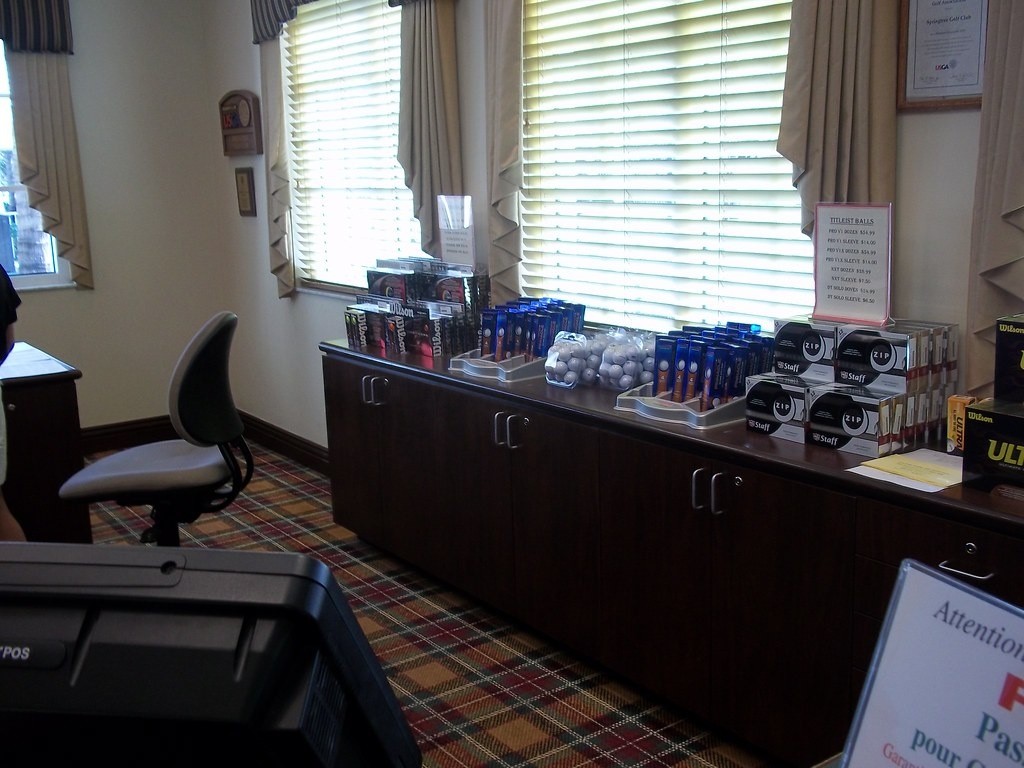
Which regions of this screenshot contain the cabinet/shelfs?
[316,312,1024,768]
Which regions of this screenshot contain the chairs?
[57,309,253,547]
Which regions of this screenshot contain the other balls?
[484,296,768,410]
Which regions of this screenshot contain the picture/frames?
[896,1,992,110]
